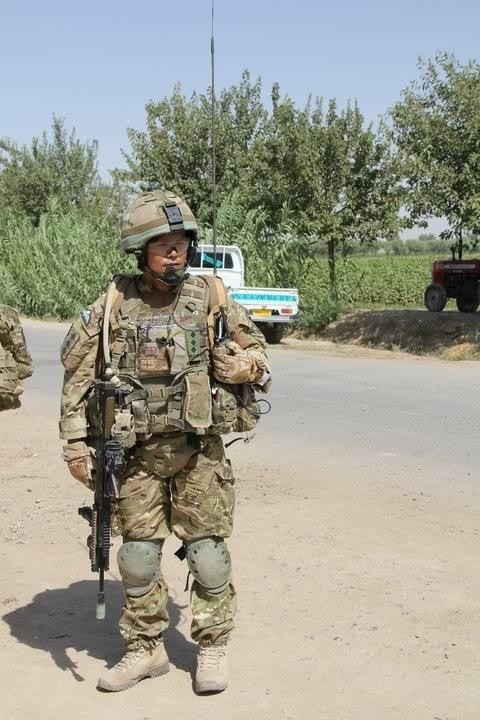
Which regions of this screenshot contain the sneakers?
[195,641,228,693]
[98,642,170,691]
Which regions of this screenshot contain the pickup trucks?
[186,245,300,345]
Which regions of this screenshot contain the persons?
[60,189,271,693]
[1,304,35,411]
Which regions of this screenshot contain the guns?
[79,381,127,620]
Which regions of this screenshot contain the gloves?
[62,439,95,494]
[211,341,264,385]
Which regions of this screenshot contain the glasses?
[144,240,190,258]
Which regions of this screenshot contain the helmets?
[120,190,199,254]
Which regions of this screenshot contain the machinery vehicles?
[425,259,480,314]
